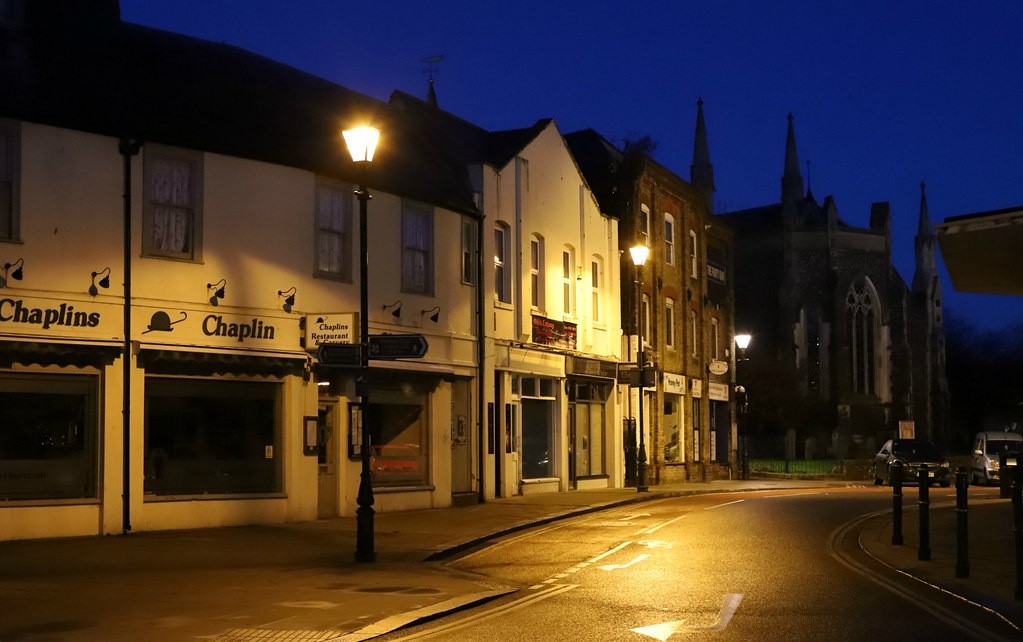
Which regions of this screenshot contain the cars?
[872,439,954,487]
[840,450,873,480]
[370,444,421,483]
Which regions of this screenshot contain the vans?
[970,430,1023,487]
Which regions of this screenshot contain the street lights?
[627,238,652,492]
[337,117,382,565]
[734,333,753,480]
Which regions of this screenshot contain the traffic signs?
[316,343,362,368]
[368,333,428,358]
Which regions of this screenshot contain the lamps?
[421,306,441,323]
[92,267,111,288]
[383,301,402,318]
[277,286,297,306]
[207,279,227,299]
[4,258,24,281]
